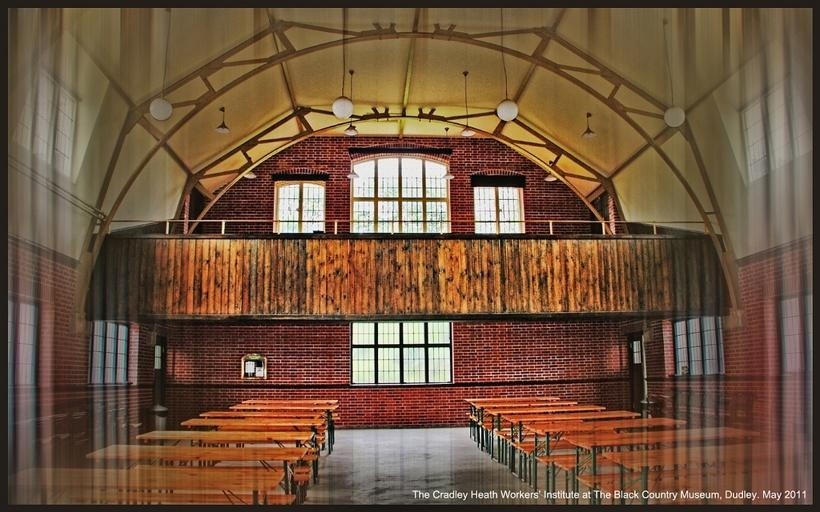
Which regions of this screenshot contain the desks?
[8,398,340,505]
[462,396,813,504]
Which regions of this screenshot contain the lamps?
[493,9,520,122]
[330,6,357,138]
[150,8,174,122]
[579,111,598,141]
[459,71,475,138]
[214,106,231,134]
[656,20,686,129]
